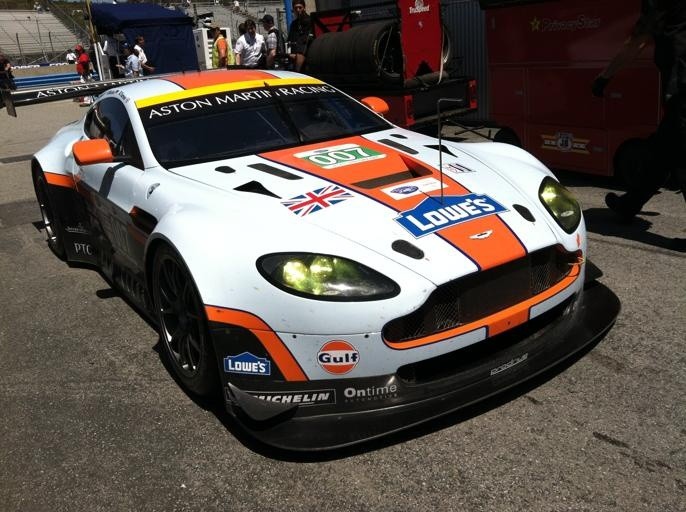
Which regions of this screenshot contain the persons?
[606,0,686,251]
[233,19,265,69]
[211,27,234,70]
[262,15,288,70]
[102,35,155,78]
[0,54,17,117]
[66,49,76,65]
[286,0,315,73]
[75,44,93,107]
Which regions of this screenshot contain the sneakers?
[606,192,636,222]
[80,103,91,107]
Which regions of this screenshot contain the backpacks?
[268,29,286,57]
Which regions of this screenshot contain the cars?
[32,68,586,452]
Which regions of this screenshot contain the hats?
[258,15,273,23]
[208,22,220,28]
[121,43,129,49]
[74,45,82,51]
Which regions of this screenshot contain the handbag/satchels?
[257,54,266,65]
